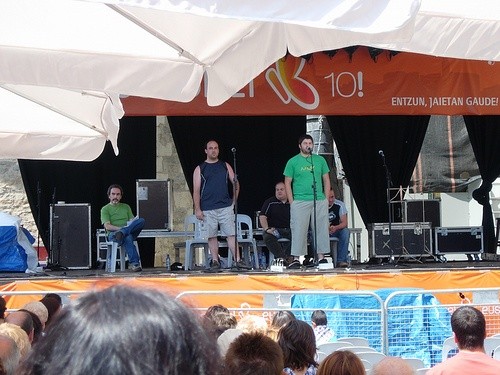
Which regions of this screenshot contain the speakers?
[50,203,92,270]
[136,178,174,232]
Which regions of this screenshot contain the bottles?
[166,254,170,268]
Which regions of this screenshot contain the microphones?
[232,147,236,152]
[379,150,384,157]
[307,147,312,151]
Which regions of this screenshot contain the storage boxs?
[50,203,92,269]
[96,229,129,261]
[402,199,442,229]
[136,178,174,231]
[434,226,484,254]
[368,222,432,258]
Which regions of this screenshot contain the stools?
[269,238,290,266]
[105,241,141,273]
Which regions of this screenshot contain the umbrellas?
[0,1,500,162]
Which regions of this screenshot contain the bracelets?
[265,228,271,234]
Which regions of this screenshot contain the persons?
[99,183,148,274]
[282,133,333,270]
[306,185,351,268]
[0,281,366,375]
[259,180,301,269]
[423,304,500,375]
[191,138,254,274]
[370,355,413,375]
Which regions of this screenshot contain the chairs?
[185,214,259,271]
[314,334,500,375]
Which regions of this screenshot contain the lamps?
[301,46,400,63]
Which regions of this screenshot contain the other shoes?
[319,259,328,263]
[209,257,222,272]
[337,262,348,267]
[286,256,293,267]
[232,257,251,268]
[289,260,300,269]
[128,264,142,272]
[115,231,124,241]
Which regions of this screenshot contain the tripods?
[365,160,443,268]
[217,154,253,272]
